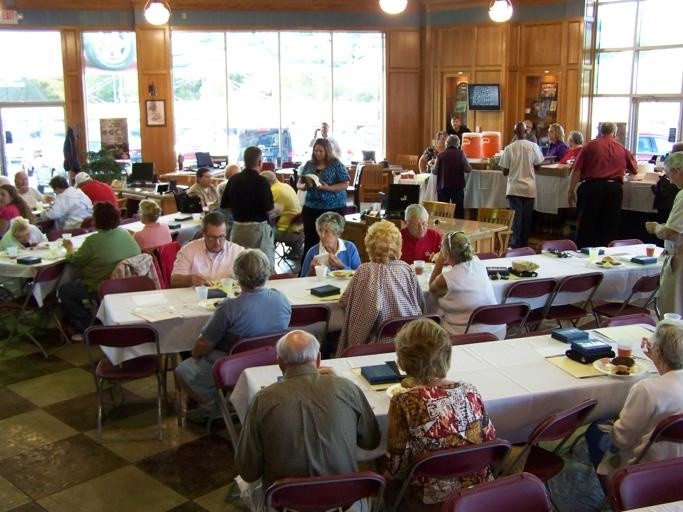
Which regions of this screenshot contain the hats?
[74,172,90,188]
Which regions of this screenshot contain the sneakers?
[72,334,83,341]
[186,408,209,422]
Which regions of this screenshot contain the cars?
[622,132,671,162]
[241,125,290,167]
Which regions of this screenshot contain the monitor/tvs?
[389,184,420,221]
[132,163,153,185]
[195,152,213,168]
[467,83,501,112]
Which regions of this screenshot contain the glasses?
[448,231,464,251]
[207,234,225,240]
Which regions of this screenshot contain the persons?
[584,318,683,494]
[382,316,498,505]
[334,218,426,359]
[428,231,507,340]
[186,147,303,274]
[651,143,683,247]
[174,248,293,425]
[398,203,443,262]
[646,150,683,320]
[498,118,639,253]
[170,213,242,286]
[235,330,382,504]
[1,171,172,340]
[296,123,351,276]
[419,111,473,219]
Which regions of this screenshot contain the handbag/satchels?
[178,192,203,213]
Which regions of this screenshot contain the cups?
[191,213,200,225]
[315,265,327,282]
[414,260,425,275]
[589,247,600,261]
[35,201,42,211]
[7,246,17,256]
[49,242,58,257]
[222,278,233,287]
[617,344,631,357]
[646,244,656,257]
[62,233,72,239]
[195,286,208,300]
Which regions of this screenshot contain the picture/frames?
[145,98,167,128]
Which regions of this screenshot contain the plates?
[328,270,355,280]
[593,261,624,268]
[593,358,644,377]
[663,312,681,320]
[197,298,223,311]
[386,382,403,397]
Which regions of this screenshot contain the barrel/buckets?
[462,132,482,162]
[479,131,501,163]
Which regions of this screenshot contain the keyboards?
[128,183,155,187]
[389,213,402,219]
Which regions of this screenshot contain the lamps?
[143,0,172,27]
